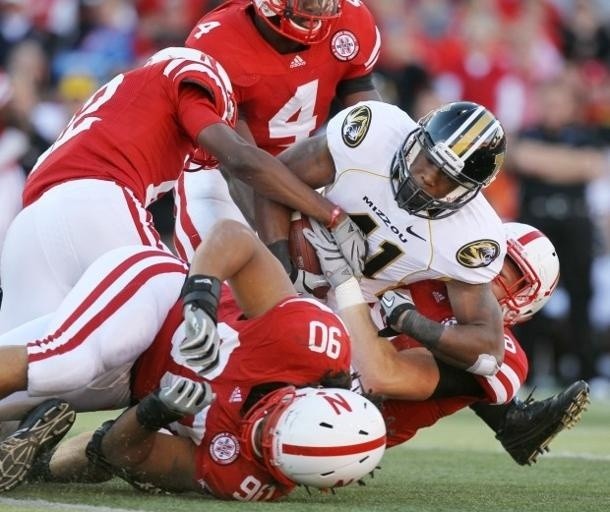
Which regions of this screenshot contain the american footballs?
[288,211,329,298]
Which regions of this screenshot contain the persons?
[3,0,610,384]
[3,1,588,500]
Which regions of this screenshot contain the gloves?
[332,217,368,280]
[302,216,364,287]
[380,283,415,329]
[269,239,298,284]
[181,276,221,374]
[140,372,215,431]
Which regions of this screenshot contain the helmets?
[255,1,341,46]
[489,223,558,325]
[393,103,504,221]
[144,46,239,173]
[238,385,387,490]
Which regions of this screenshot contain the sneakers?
[84,420,179,496]
[497,379,590,465]
[0,399,77,494]
